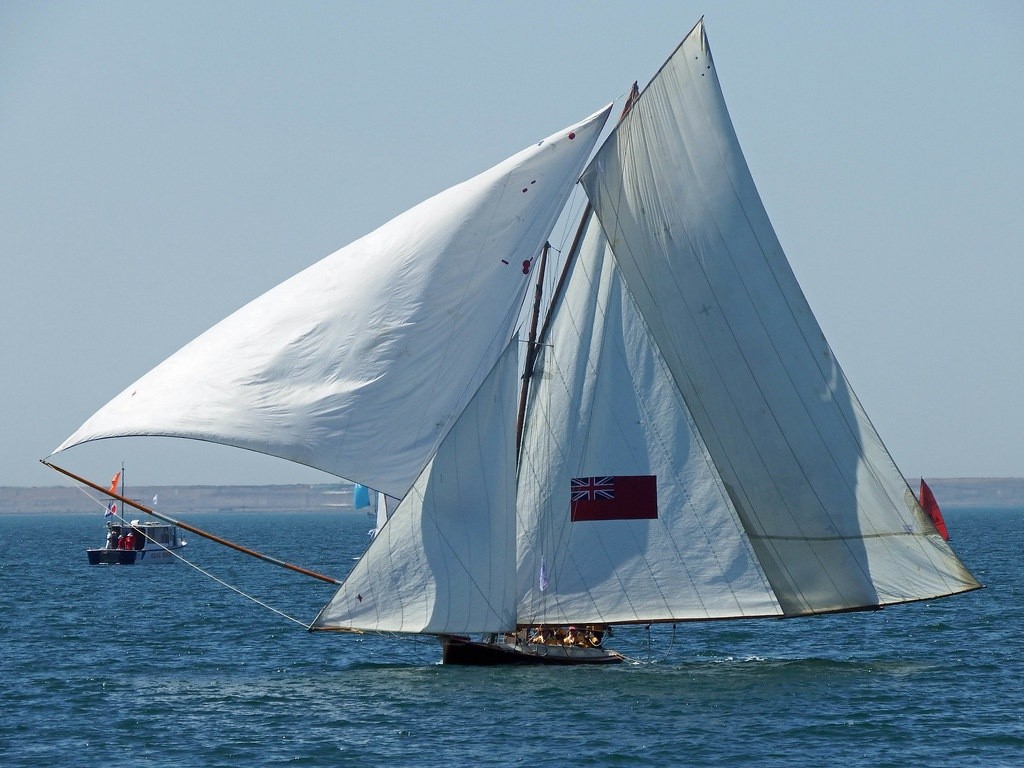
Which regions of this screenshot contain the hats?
[118,535,124,540]
[569,626,576,631]
[127,533,133,537]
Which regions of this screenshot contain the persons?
[532,624,612,648]
[118,532,136,550]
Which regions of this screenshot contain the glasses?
[108,538,111,540]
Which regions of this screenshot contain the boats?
[87,459,187,565]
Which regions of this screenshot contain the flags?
[539,559,548,590]
[152,495,158,505]
[920,478,948,541]
[571,477,658,519]
[109,472,120,493]
[104,503,116,517]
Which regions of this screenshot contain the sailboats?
[36,9,988,670]
